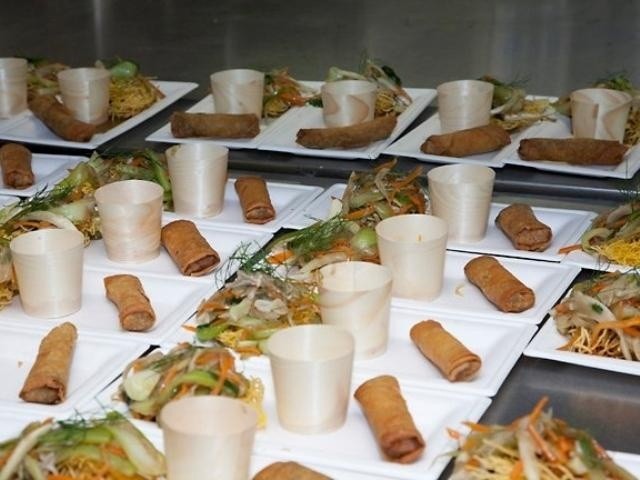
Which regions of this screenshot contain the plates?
[26,166,323,233]
[144,75,324,149]
[1,146,90,199]
[168,275,537,397]
[0,325,152,415]
[451,450,640,480]
[1,78,198,151]
[525,284,640,375]
[562,213,640,275]
[2,267,226,347]
[502,101,640,179]
[381,93,556,170]
[228,233,583,323]
[1,401,64,450]
[282,182,598,263]
[254,83,438,160]
[79,347,494,480]
[77,222,274,282]
[60,419,400,480]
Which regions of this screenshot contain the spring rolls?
[296,113,398,148]
[17,322,79,406]
[162,218,220,273]
[410,319,480,380]
[31,93,94,142]
[517,135,629,168]
[234,175,273,220]
[103,273,154,329]
[254,461,328,479]
[421,126,511,155]
[168,112,266,140]
[2,144,41,188]
[495,201,550,250]
[464,255,535,313]
[355,375,425,463]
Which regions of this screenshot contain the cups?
[210,68,264,122]
[375,213,449,301]
[56,68,111,126]
[428,163,496,244]
[269,324,356,434]
[94,179,164,264]
[570,89,634,143]
[320,79,377,128]
[10,228,85,319]
[165,143,230,220]
[159,393,258,480]
[437,79,495,134]
[315,261,393,360]
[1,56,28,118]
